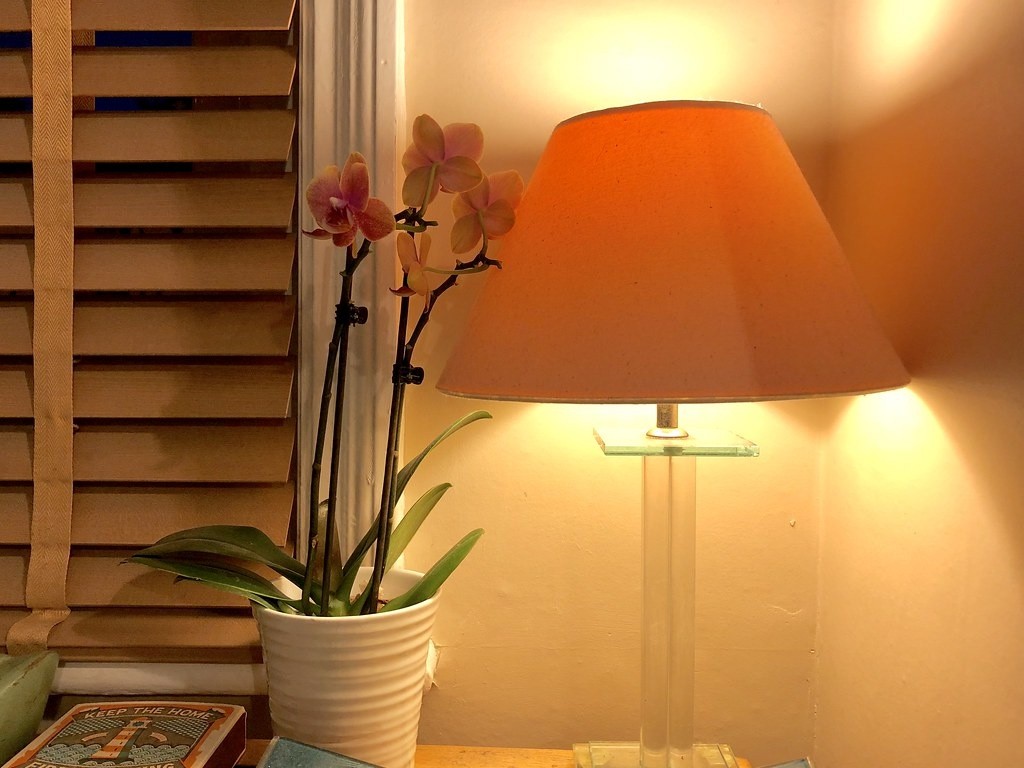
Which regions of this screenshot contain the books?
[0,701,247,768]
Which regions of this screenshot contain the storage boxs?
[0,700,246,768]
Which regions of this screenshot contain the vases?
[244,563,436,768]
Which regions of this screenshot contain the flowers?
[118,109,521,603]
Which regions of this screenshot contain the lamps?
[434,96,916,767]
